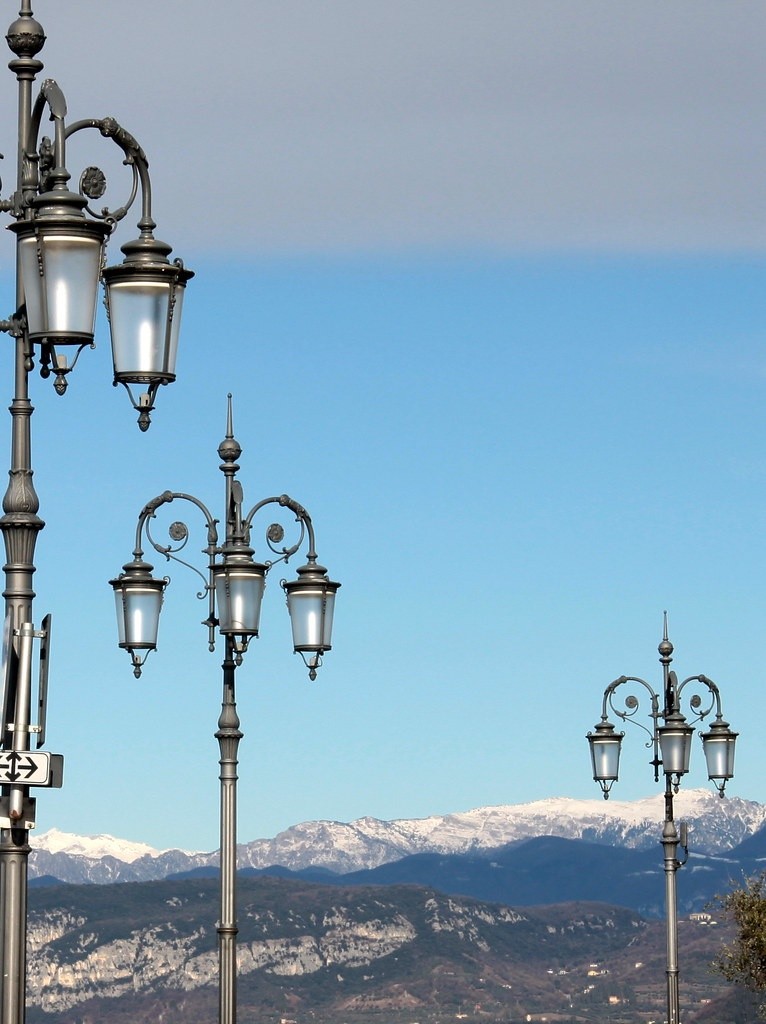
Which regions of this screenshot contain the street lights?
[109,391,343,1024]
[587,610,742,1024]
[0,2,196,1024]
